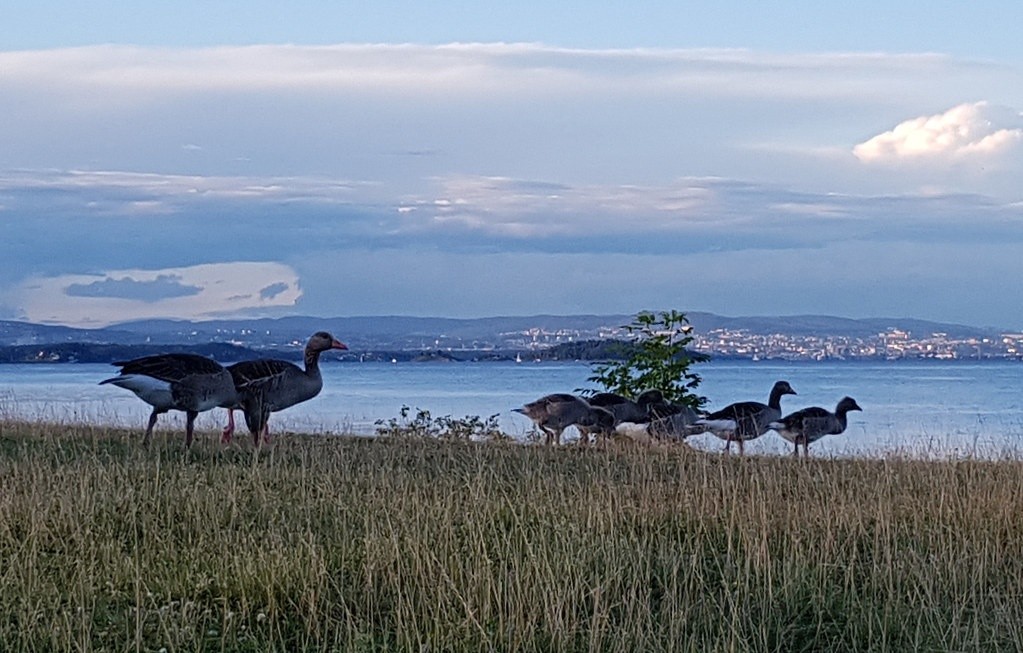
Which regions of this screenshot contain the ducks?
[509,380,863,457]
[99,329,350,451]
